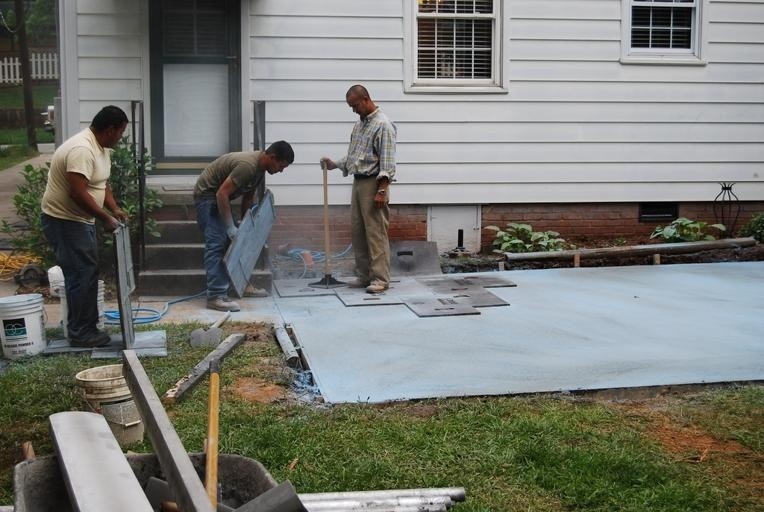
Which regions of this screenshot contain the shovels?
[190,308,230,350]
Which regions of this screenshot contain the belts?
[354,173,374,180]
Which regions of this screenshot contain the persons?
[192,139,295,312]
[39,103,129,346]
[318,84,397,296]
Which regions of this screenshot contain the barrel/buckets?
[59,281,105,336]
[75,364,145,446]
[0,293,49,360]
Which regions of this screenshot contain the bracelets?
[377,188,388,195]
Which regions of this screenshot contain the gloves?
[225,225,239,241]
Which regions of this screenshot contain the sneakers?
[68,328,111,348]
[347,279,368,289]
[206,297,239,312]
[355,281,387,295]
[228,282,268,298]
